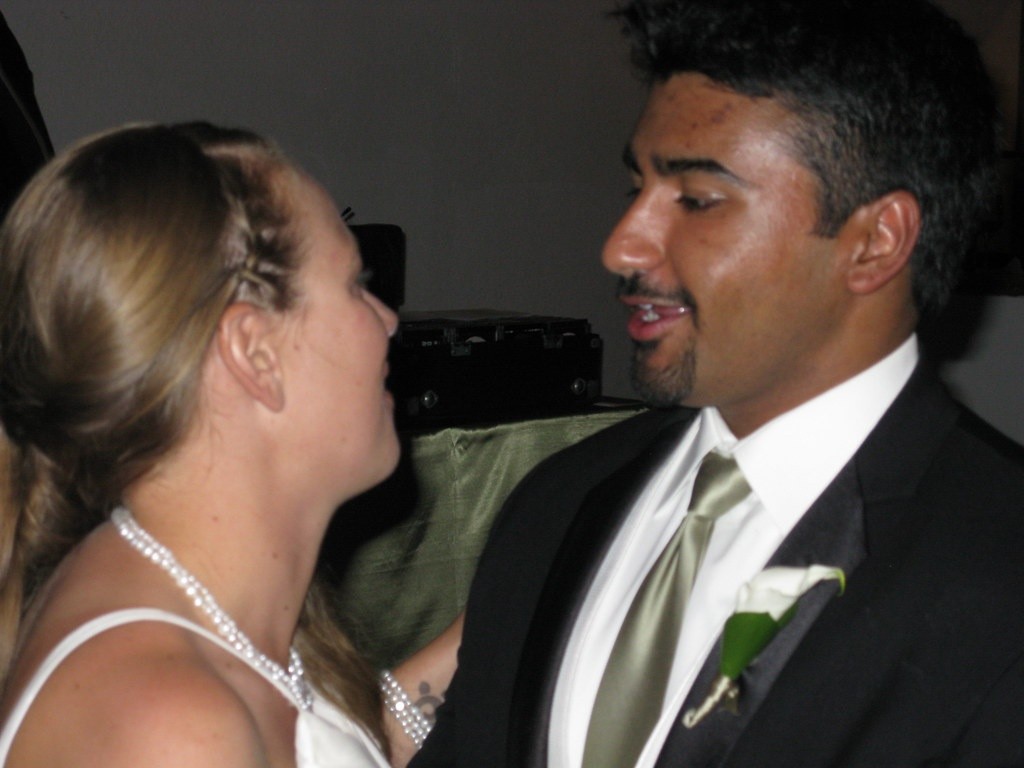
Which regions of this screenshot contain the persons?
[0,121,466,768]
[0,11,60,566]
[405,0,1023,768]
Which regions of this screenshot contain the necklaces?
[109,506,314,716]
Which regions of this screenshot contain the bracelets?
[375,670,432,752]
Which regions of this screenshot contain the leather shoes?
[582,451,752,768]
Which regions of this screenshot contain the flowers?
[684,565,847,729]
